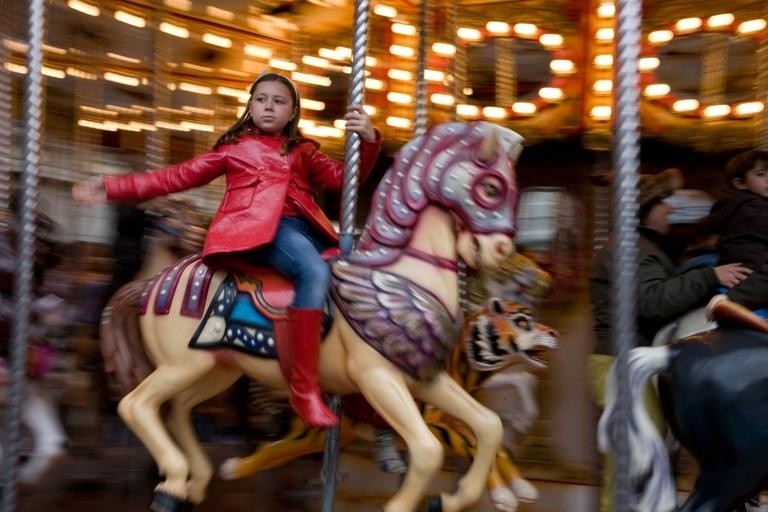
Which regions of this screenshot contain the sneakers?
[370,445,408,475]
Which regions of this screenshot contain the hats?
[609,166,682,231]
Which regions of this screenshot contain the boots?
[283,306,341,430]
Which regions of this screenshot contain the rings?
[733,279,738,284]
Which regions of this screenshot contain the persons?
[589,167,754,512]
[711,150,768,319]
[71,73,383,430]
